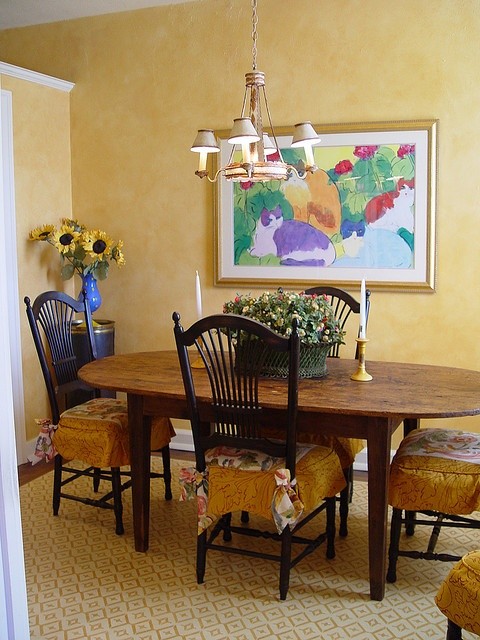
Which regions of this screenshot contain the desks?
[46,318,117,411]
[74,348,480,601]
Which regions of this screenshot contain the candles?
[194,271,202,321]
[357,278,368,339]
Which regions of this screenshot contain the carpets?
[18,455,478,640]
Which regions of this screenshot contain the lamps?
[187,0,322,185]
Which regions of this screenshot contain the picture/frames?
[205,118,441,295]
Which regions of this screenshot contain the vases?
[74,272,104,329]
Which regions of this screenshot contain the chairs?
[23,288,174,537]
[170,310,349,602]
[387,427,480,585]
[274,283,371,539]
[432,549,479,640]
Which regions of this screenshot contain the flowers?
[27,218,125,282]
[214,291,348,347]
